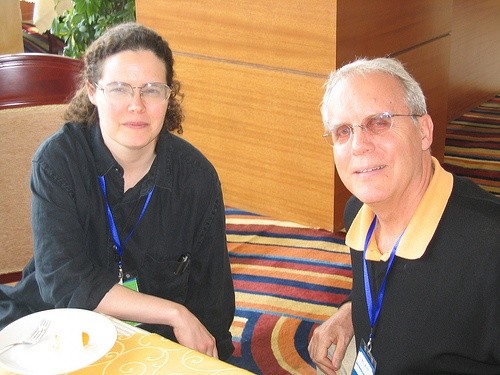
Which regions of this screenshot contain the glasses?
[94,82,172,103]
[322,111,422,147]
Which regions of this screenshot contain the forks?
[0,320,50,356]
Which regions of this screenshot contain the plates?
[0,308,117,374]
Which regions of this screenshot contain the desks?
[0,311,259,374]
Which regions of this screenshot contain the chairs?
[0,53,93,283]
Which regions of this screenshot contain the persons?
[306,56,500,375]
[0,23,236,365]
